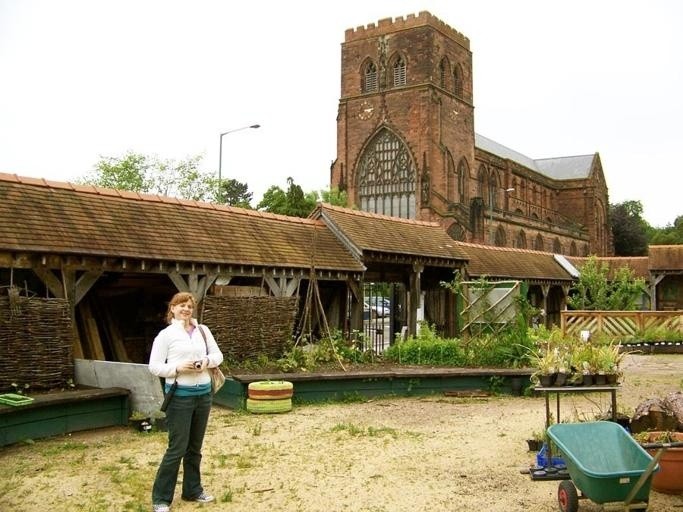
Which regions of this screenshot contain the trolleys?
[546,422,660,512]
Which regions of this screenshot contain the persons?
[147,291,224,512]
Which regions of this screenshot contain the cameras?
[192,359,203,370]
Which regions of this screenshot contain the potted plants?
[516,331,629,387]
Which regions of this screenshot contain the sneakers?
[153,504,169,512]
[181,492,214,502]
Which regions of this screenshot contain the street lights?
[218,125,260,205]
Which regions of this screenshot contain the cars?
[364,296,391,320]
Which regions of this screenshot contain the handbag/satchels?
[209,367,226,394]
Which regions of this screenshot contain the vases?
[634,430,683,496]
